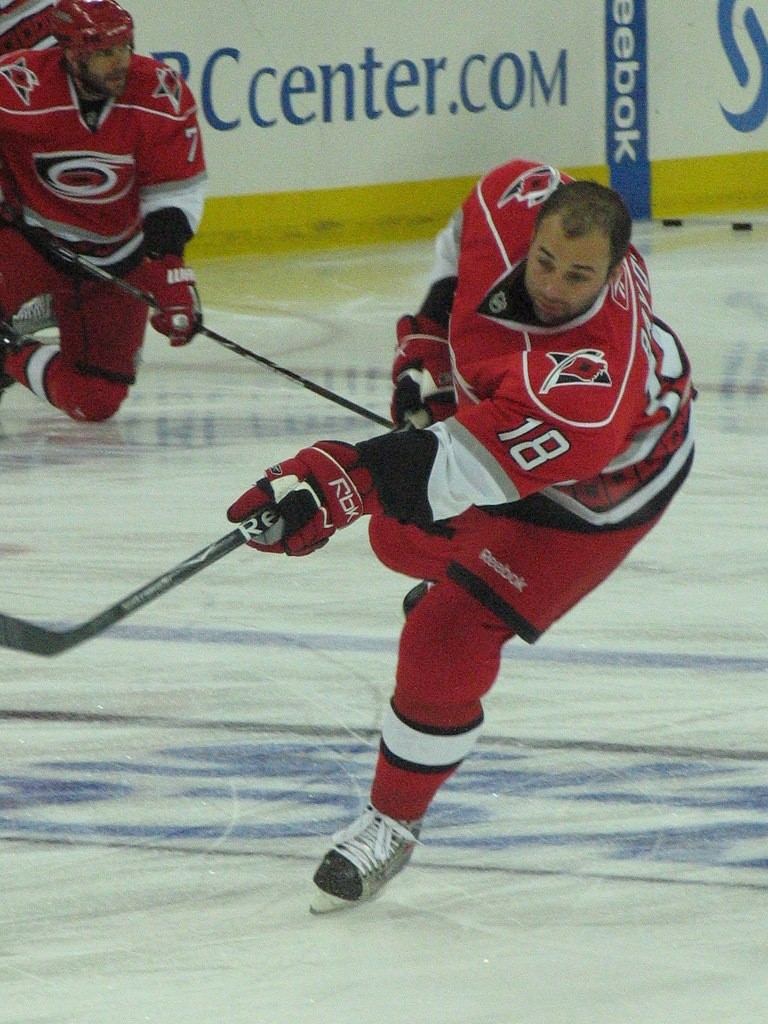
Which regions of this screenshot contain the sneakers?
[308,798,427,914]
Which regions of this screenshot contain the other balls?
[662,218,681,226]
[732,222,751,231]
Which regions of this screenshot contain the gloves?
[390,315,456,425]
[143,253,202,347]
[226,441,385,557]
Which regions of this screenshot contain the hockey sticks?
[14,220,398,431]
[0,410,431,658]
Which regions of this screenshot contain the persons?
[228,158,698,916]
[0,0,210,423]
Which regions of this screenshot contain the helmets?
[50,0,134,52]
